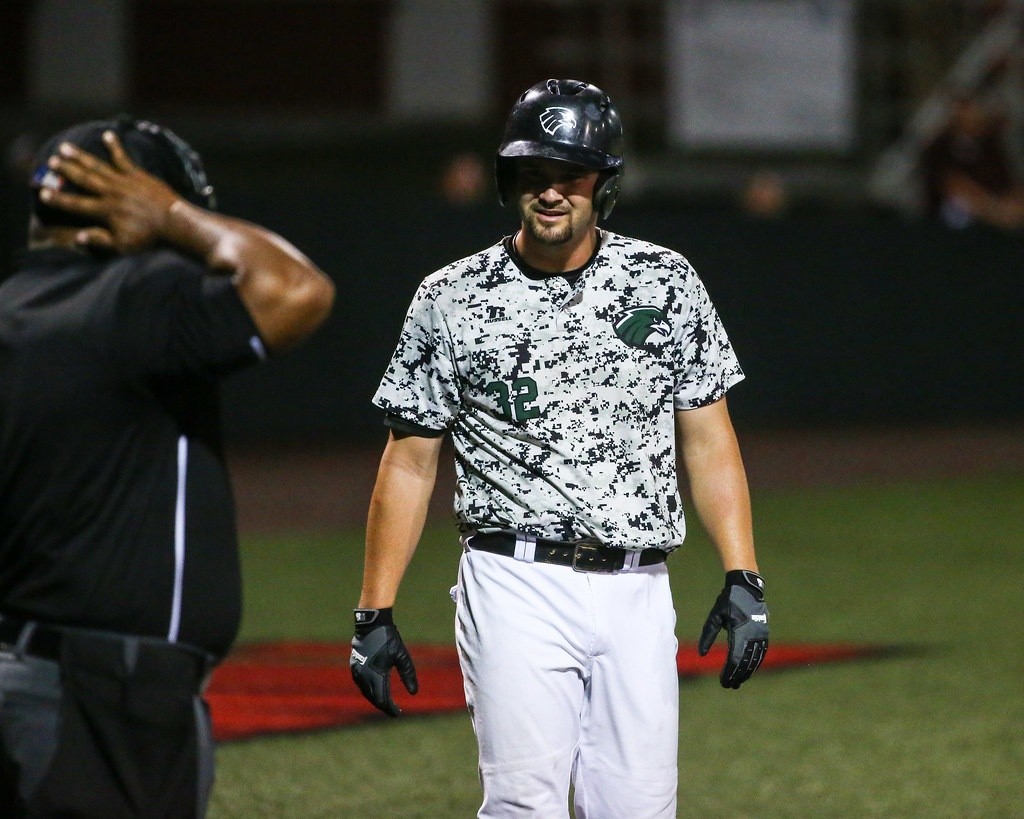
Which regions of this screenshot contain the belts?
[470,530,667,573]
[2,615,215,694]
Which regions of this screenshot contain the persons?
[352,78,769,819]
[0,123,332,819]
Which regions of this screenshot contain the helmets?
[493,80,624,220]
[29,116,216,264]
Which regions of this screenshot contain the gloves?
[698,570,769,689]
[349,608,418,718]
